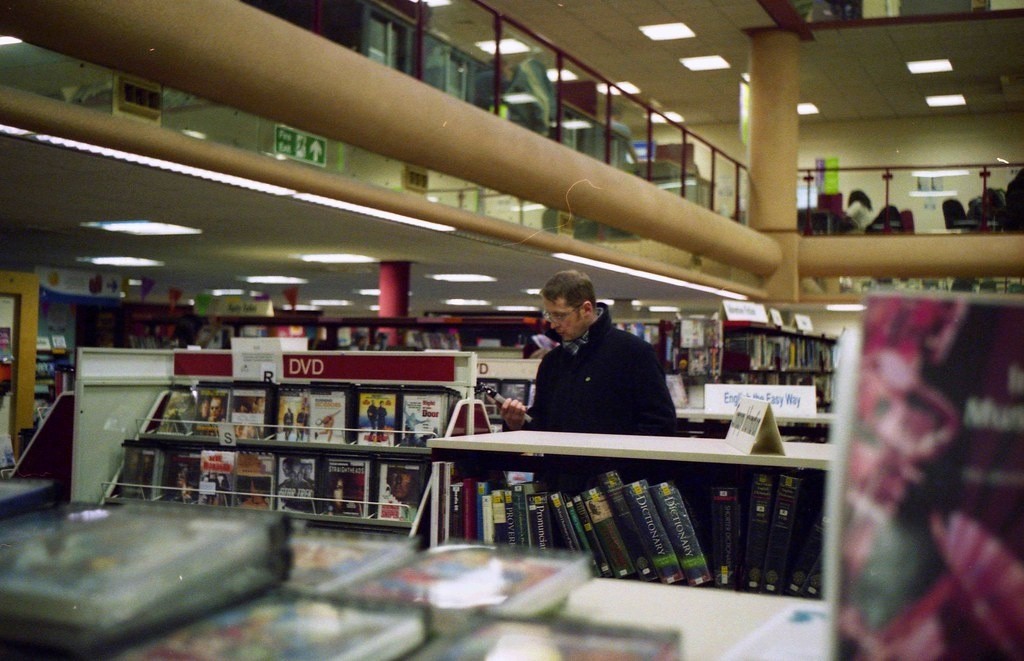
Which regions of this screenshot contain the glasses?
[542,302,583,324]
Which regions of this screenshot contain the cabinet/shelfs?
[0,310,838,661]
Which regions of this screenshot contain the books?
[620,319,834,442]
[127,391,457,534]
[429,460,823,599]
[33,361,77,424]
[0,502,682,660]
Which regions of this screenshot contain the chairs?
[799,187,1010,236]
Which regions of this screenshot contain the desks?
[559,100,631,168]
[634,157,712,210]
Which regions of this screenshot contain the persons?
[207,397,224,422]
[377,401,387,441]
[277,457,314,513]
[845,191,875,235]
[296,407,307,442]
[367,401,376,441]
[495,269,676,434]
[371,332,387,351]
[283,409,293,440]
[301,463,314,486]
[348,335,366,351]
